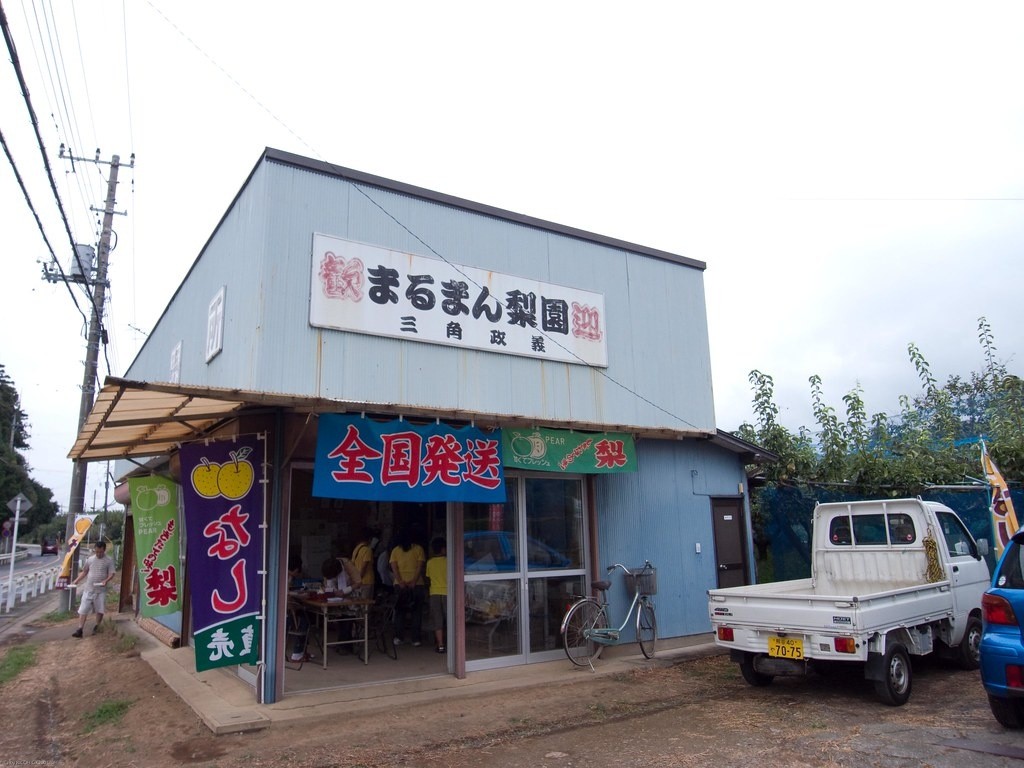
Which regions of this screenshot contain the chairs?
[284,601,323,671]
[350,591,402,662]
[832,527,857,544]
[893,524,914,544]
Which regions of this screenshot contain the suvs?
[455,530,570,577]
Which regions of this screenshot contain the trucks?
[707,495,992,707]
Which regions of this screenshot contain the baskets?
[624,568,657,595]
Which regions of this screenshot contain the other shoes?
[393,637,401,645]
[415,642,421,646]
[336,643,353,649]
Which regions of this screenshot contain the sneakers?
[291,652,315,660]
[434,646,445,653]
[72,630,82,637]
[92,626,97,634]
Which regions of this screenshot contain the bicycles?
[561,558,662,675]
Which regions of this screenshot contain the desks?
[287,589,377,670]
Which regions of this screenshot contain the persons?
[426,537,448,653]
[68,541,116,638]
[389,526,425,646]
[285,554,315,661]
[377,537,396,596]
[351,530,375,639]
[322,557,363,655]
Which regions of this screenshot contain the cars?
[976,524,1024,728]
[40,538,58,556]
